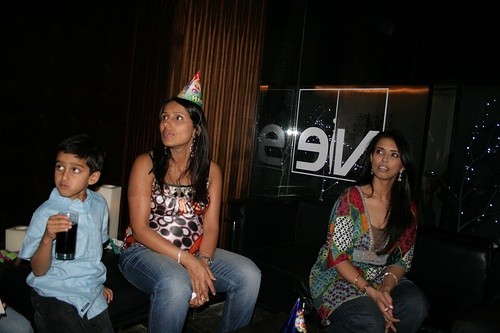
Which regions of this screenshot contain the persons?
[309,129,429,333]
[117,70,261,333]
[19,133,112,333]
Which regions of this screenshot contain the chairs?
[118,263,224,333]
[293,200,334,333]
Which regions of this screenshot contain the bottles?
[284,296,315,333]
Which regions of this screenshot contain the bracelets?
[381,271,398,286]
[362,284,374,297]
[195,251,213,266]
[352,274,364,289]
[177,248,184,264]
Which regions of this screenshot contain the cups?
[54,211,79,261]
